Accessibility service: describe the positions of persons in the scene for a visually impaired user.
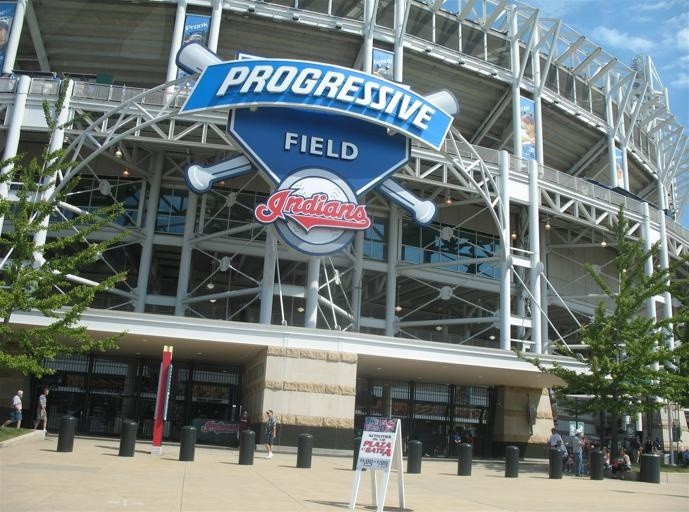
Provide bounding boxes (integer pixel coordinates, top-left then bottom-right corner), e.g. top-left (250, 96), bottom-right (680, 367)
top-left (683, 448), bottom-right (689, 463)
top-left (545, 428), bottom-right (563, 450)
top-left (581, 435), bottom-right (590, 458)
top-left (264, 409), bottom-right (276, 457)
top-left (1, 389), bottom-right (23, 430)
top-left (466, 433), bottom-right (474, 444)
top-left (453, 431), bottom-right (461, 458)
top-left (564, 448), bottom-right (574, 472)
top-left (561, 435), bottom-right (569, 469)
top-left (34, 388), bottom-right (51, 433)
top-left (592, 434), bottom-right (662, 472)
top-left (573, 430), bottom-right (585, 476)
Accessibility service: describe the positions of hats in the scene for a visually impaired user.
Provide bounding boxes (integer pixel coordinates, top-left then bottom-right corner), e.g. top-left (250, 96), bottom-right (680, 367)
top-left (574, 430), bottom-right (582, 436)
